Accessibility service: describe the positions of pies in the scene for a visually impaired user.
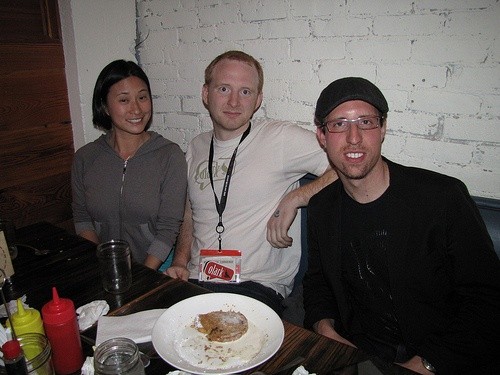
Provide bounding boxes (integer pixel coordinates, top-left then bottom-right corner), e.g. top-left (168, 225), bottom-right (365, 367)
top-left (198, 310), bottom-right (248, 343)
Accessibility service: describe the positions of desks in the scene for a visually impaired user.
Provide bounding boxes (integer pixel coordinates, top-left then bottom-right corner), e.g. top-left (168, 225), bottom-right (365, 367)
top-left (0, 220), bottom-right (428, 375)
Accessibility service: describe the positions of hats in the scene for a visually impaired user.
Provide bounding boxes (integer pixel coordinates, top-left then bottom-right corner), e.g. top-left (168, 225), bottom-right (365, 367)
top-left (314, 76), bottom-right (388, 125)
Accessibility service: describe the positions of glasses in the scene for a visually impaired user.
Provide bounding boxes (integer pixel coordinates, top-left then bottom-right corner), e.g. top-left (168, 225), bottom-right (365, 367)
top-left (319, 116), bottom-right (380, 133)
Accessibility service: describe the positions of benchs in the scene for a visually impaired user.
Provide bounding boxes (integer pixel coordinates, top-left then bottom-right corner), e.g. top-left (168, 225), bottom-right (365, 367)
top-left (291, 173), bottom-right (500, 328)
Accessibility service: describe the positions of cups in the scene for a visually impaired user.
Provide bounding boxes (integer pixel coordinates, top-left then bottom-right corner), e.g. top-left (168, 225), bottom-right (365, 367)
top-left (0, 223), bottom-right (17, 259)
top-left (96, 239), bottom-right (132, 294)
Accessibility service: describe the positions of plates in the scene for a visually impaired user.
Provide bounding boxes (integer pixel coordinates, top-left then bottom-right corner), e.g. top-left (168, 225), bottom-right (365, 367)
top-left (151, 293), bottom-right (284, 375)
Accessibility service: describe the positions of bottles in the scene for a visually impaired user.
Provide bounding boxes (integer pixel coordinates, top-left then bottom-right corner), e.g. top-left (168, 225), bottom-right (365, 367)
top-left (6, 299), bottom-right (55, 375)
top-left (41, 287), bottom-right (83, 375)
top-left (14, 333), bottom-right (55, 375)
top-left (94, 337), bottom-right (144, 375)
top-left (2, 340), bottom-right (28, 375)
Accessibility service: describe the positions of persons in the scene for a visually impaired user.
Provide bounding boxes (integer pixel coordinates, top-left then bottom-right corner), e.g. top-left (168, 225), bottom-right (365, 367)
top-left (163, 50), bottom-right (339, 308)
top-left (70, 59), bottom-right (187, 272)
top-left (302, 76), bottom-right (500, 375)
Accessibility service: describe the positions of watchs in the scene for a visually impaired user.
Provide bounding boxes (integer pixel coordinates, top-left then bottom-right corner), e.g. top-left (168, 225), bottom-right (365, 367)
top-left (422, 358), bottom-right (437, 373)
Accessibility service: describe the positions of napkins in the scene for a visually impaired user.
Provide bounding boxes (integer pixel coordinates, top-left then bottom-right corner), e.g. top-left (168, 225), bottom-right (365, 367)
top-left (93, 306), bottom-right (167, 349)
